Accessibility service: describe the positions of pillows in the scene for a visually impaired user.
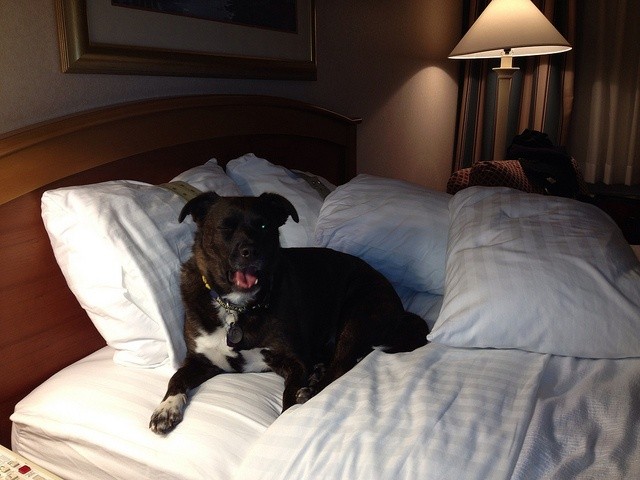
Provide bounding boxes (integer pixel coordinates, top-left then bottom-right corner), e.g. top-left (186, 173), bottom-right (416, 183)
top-left (226, 152), bottom-right (337, 250)
top-left (425, 183), bottom-right (640, 360)
top-left (317, 171), bottom-right (456, 297)
top-left (37, 155), bottom-right (245, 371)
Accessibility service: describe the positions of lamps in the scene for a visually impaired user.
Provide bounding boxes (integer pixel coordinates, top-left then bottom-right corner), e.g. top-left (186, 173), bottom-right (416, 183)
top-left (446, 0), bottom-right (574, 163)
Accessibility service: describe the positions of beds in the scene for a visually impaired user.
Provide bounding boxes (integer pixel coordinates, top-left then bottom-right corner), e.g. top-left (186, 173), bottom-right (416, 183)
top-left (2, 96), bottom-right (640, 480)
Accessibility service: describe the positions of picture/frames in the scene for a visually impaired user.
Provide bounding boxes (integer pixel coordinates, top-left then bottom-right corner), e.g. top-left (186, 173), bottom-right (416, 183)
top-left (53, 1), bottom-right (319, 84)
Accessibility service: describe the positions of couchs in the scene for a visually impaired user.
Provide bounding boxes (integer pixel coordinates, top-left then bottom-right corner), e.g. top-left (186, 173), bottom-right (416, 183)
top-left (448, 152), bottom-right (583, 197)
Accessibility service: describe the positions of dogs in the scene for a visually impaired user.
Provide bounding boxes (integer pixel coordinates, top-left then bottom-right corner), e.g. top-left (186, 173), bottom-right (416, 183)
top-left (150, 190), bottom-right (429, 436)
